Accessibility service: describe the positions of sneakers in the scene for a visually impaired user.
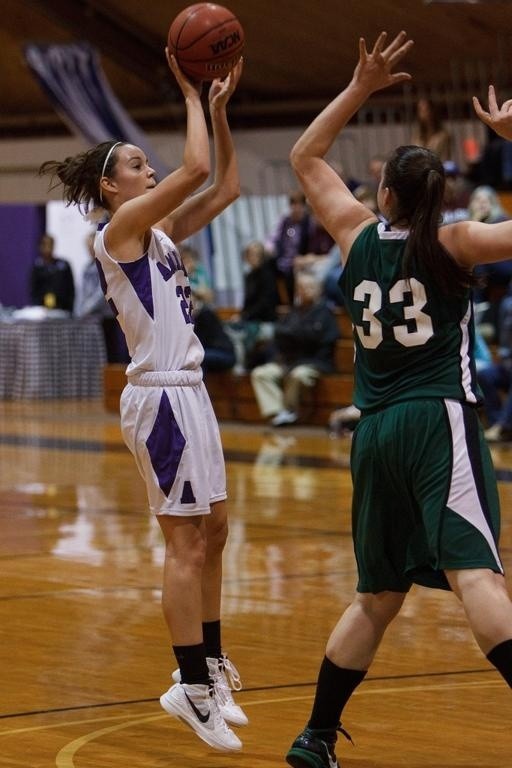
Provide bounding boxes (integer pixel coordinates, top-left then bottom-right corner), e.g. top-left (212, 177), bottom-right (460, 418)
top-left (270, 409), bottom-right (297, 426)
top-left (161, 682), bottom-right (243, 755)
top-left (173, 654), bottom-right (247, 727)
top-left (285, 727), bottom-right (337, 767)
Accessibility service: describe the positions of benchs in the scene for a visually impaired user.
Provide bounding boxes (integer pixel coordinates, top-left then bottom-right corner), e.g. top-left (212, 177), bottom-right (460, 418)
top-left (103, 309), bottom-right (510, 424)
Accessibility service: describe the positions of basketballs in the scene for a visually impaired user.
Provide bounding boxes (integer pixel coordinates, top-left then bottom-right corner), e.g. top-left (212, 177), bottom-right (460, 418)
top-left (169, 2), bottom-right (244, 83)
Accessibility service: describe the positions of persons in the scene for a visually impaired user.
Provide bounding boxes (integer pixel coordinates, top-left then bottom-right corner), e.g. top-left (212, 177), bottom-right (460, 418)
top-left (281, 23), bottom-right (512, 767)
top-left (30, 234), bottom-right (74, 314)
top-left (64, 43), bottom-right (252, 756)
top-left (177, 98), bottom-right (511, 445)
